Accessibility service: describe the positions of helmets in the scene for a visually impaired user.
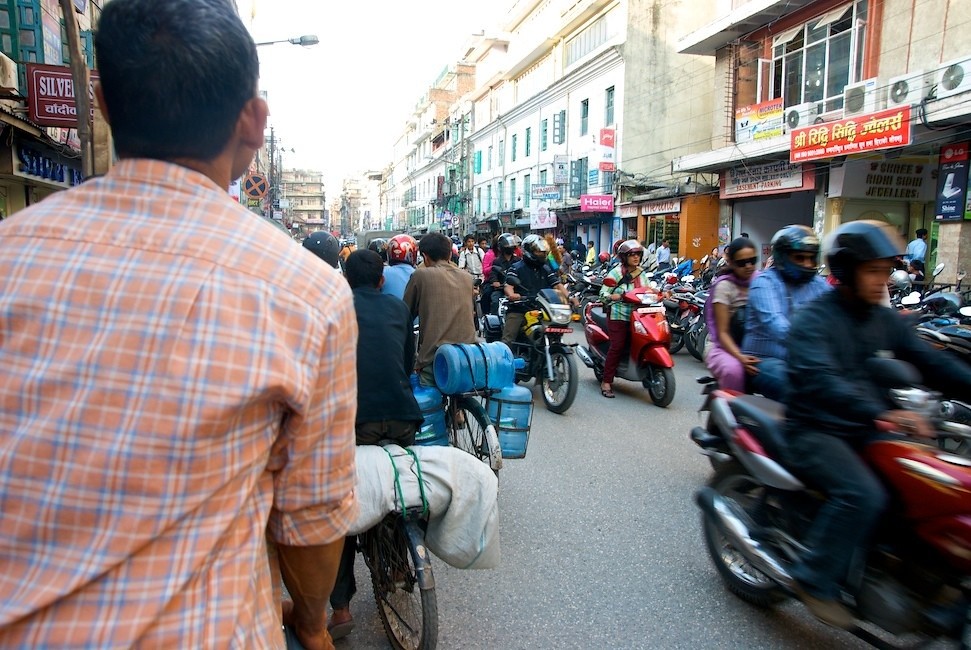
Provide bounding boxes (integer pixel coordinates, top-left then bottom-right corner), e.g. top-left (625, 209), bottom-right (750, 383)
top-left (369, 238), bottom-right (388, 262)
top-left (451, 235), bottom-right (459, 242)
top-left (570, 251), bottom-right (581, 259)
top-left (347, 238), bottom-right (355, 245)
top-left (618, 241), bottom-right (644, 263)
top-left (497, 233), bottom-right (516, 255)
top-left (824, 219), bottom-right (910, 287)
top-left (302, 230), bottom-right (339, 269)
top-left (521, 234), bottom-right (546, 265)
top-left (770, 224), bottom-right (821, 286)
top-left (387, 234), bottom-right (417, 267)
top-left (514, 235), bottom-right (523, 246)
top-left (598, 252), bottom-right (611, 262)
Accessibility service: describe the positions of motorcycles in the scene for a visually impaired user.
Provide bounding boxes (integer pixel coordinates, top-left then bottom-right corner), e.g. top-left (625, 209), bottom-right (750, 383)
top-left (635, 254), bottom-right (720, 360)
top-left (562, 251), bottom-right (621, 313)
top-left (890, 263), bottom-right (971, 354)
top-left (690, 353), bottom-right (971, 650)
top-left (484, 274), bottom-right (582, 416)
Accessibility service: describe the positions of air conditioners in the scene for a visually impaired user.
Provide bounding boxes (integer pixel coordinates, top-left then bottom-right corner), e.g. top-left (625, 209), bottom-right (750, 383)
top-left (843, 77), bottom-right (882, 118)
top-left (0, 52), bottom-right (20, 95)
top-left (785, 102), bottom-right (818, 133)
top-left (936, 56), bottom-right (971, 99)
top-left (888, 70), bottom-right (934, 108)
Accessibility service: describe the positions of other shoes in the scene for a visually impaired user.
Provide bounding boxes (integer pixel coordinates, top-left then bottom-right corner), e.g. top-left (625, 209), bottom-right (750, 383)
top-left (793, 582), bottom-right (855, 632)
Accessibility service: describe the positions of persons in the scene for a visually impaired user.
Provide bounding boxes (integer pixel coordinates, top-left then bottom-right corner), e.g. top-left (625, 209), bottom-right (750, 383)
top-left (302, 229), bottom-right (930, 549)
top-left (328, 249), bottom-right (425, 634)
top-left (0, 0), bottom-right (357, 650)
top-left (786, 219), bottom-right (971, 630)
top-left (741, 224), bottom-right (835, 403)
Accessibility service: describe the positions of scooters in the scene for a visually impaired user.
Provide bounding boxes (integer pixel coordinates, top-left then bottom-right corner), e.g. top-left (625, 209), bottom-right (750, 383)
top-left (576, 273), bottom-right (679, 408)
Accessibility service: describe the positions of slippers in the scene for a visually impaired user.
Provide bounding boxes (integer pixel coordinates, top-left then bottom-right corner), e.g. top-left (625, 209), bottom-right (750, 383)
top-left (327, 620), bottom-right (356, 641)
top-left (601, 387), bottom-right (615, 398)
top-left (445, 409), bottom-right (465, 431)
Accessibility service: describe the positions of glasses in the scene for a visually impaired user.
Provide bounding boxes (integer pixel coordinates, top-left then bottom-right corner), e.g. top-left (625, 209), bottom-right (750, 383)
top-left (795, 254), bottom-right (816, 263)
top-left (627, 253), bottom-right (642, 257)
top-left (731, 256), bottom-right (757, 268)
top-left (860, 265), bottom-right (893, 277)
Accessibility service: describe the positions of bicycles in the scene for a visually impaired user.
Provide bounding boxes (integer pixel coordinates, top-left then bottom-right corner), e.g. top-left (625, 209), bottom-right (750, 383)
top-left (404, 324), bottom-right (534, 491)
top-left (339, 439), bottom-right (438, 650)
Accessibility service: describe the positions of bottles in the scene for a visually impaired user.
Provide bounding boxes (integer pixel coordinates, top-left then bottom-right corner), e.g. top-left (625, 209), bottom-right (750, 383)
top-left (410, 374), bottom-right (449, 446)
top-left (483, 383), bottom-right (532, 456)
top-left (433, 341), bottom-right (525, 395)
